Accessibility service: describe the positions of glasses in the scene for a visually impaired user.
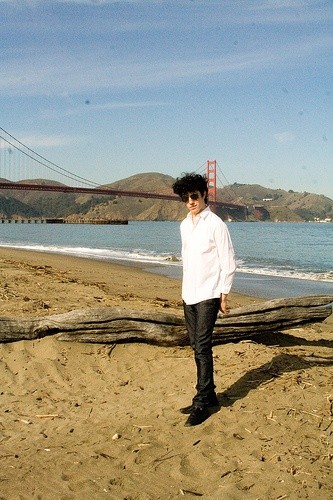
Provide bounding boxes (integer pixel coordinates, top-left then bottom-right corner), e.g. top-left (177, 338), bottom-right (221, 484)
top-left (182, 193), bottom-right (200, 202)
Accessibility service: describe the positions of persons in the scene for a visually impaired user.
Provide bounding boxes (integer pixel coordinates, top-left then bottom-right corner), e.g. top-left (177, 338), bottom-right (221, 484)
top-left (170, 173), bottom-right (237, 426)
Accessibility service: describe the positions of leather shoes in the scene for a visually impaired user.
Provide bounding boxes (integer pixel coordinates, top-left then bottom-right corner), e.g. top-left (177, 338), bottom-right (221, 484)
top-left (184, 408), bottom-right (210, 427)
top-left (180, 400), bottom-right (219, 414)
